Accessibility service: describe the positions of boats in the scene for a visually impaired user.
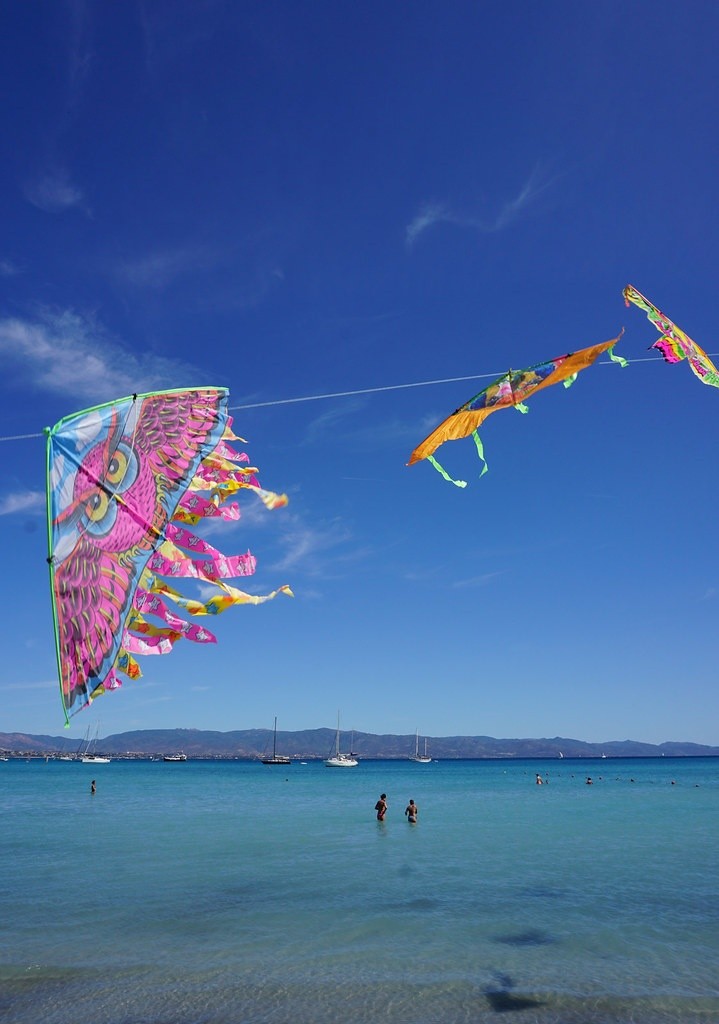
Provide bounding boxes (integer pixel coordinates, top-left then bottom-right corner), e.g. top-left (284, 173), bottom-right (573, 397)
top-left (163, 753), bottom-right (188, 761)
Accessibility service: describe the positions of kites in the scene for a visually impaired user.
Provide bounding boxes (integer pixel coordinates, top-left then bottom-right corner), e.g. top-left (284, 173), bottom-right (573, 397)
top-left (406, 326), bottom-right (632, 488)
top-left (619, 282), bottom-right (718, 392)
top-left (47, 386), bottom-right (293, 729)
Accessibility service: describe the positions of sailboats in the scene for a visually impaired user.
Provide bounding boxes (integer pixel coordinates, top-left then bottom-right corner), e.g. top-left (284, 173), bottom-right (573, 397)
top-left (407, 727), bottom-right (432, 764)
top-left (260, 716), bottom-right (291, 765)
top-left (325, 709), bottom-right (358, 767)
top-left (71, 721), bottom-right (111, 763)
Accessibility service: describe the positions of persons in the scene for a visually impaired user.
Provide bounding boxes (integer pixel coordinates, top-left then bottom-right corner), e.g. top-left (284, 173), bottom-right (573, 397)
top-left (374, 793), bottom-right (388, 821)
top-left (535, 774), bottom-right (550, 785)
top-left (586, 776), bottom-right (594, 785)
top-left (405, 799), bottom-right (419, 823)
top-left (90, 779), bottom-right (98, 796)
